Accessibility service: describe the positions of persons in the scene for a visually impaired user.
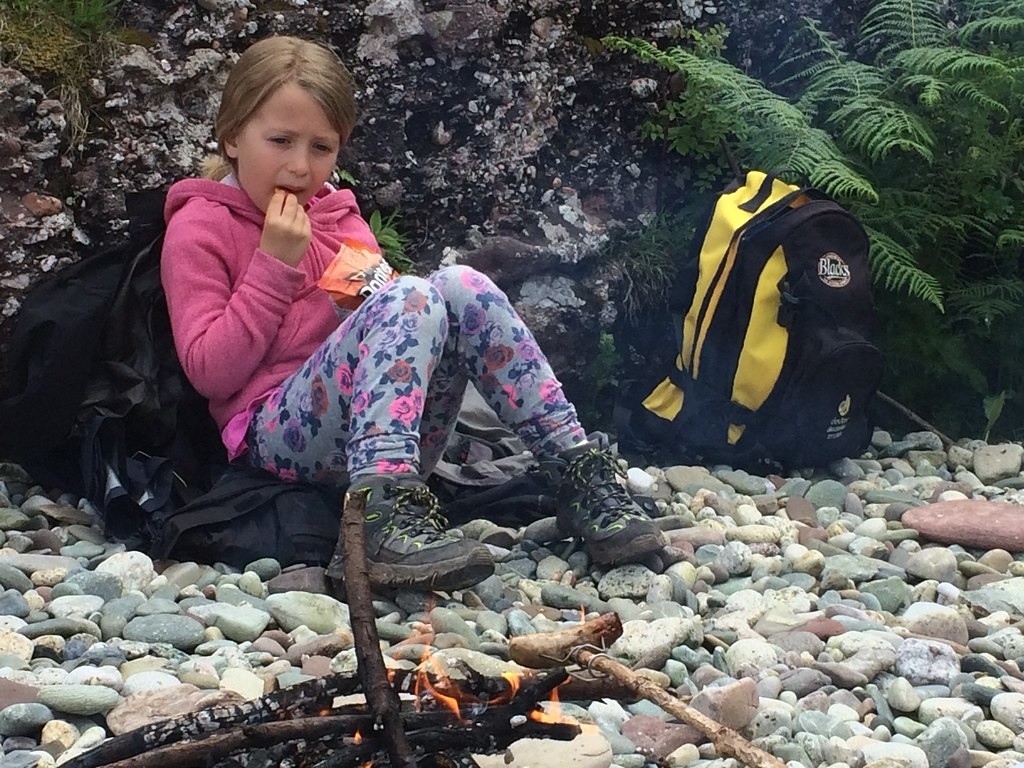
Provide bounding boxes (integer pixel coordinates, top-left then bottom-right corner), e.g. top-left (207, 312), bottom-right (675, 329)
top-left (159, 35), bottom-right (666, 598)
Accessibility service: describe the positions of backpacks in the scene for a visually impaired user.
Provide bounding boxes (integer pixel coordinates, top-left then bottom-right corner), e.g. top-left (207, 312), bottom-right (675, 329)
top-left (628, 160), bottom-right (883, 477)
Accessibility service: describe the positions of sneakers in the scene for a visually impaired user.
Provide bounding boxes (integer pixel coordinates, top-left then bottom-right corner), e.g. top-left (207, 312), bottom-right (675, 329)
top-left (324, 473), bottom-right (495, 591)
top-left (537, 431), bottom-right (667, 567)
